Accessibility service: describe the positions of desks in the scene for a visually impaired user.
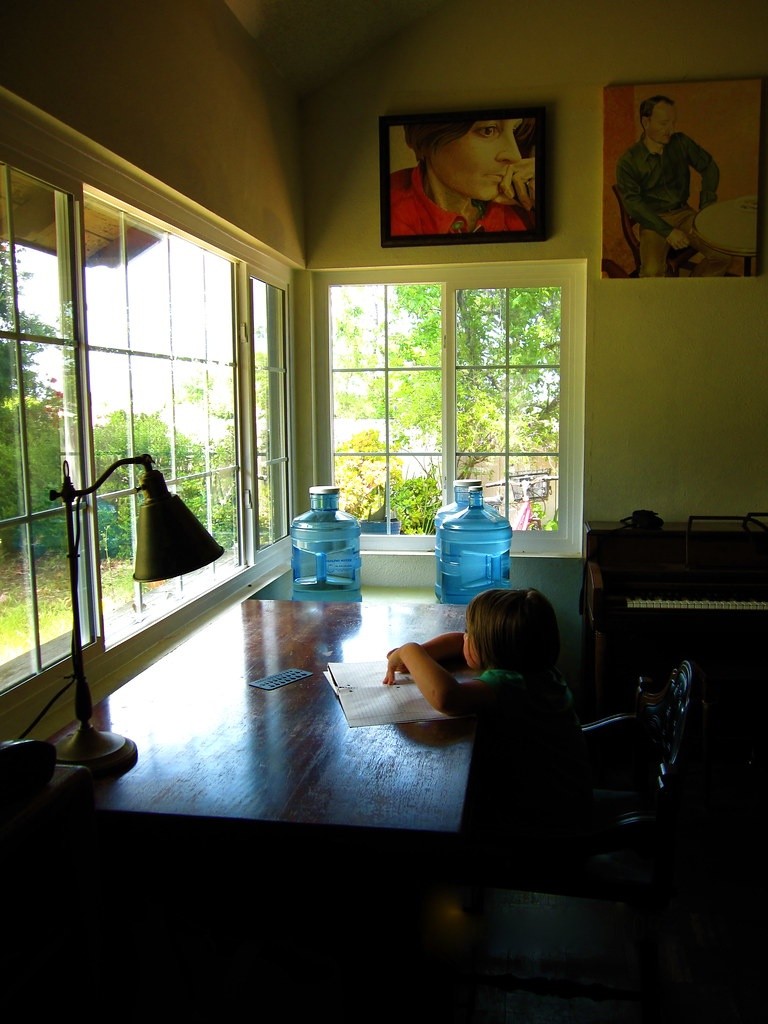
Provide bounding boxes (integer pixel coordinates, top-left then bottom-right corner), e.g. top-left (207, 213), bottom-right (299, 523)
top-left (44, 599), bottom-right (475, 835)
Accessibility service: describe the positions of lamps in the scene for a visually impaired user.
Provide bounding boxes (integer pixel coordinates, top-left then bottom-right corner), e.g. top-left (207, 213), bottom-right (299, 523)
top-left (50, 454), bottom-right (224, 777)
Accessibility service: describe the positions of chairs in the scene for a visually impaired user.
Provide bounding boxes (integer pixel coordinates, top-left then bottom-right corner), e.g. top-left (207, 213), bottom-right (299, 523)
top-left (468, 659), bottom-right (712, 1024)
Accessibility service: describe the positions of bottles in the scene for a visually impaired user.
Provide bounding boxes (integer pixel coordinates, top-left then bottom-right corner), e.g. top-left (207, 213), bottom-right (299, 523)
top-left (435, 480), bottom-right (512, 605)
top-left (291, 485), bottom-right (362, 602)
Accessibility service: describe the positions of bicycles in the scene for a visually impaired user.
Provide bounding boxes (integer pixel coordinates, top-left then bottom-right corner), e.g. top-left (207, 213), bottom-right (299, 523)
top-left (479, 466), bottom-right (559, 531)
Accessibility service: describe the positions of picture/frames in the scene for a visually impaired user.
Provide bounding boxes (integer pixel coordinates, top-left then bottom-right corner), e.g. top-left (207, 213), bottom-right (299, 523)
top-left (379, 107), bottom-right (547, 249)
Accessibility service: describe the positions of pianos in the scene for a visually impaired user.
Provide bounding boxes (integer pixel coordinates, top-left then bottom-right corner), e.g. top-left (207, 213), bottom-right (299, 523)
top-left (583, 512), bottom-right (767, 695)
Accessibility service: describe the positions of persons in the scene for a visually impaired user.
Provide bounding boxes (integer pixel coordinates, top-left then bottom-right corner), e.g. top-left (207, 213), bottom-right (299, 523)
top-left (407, 586), bottom-right (593, 826)
